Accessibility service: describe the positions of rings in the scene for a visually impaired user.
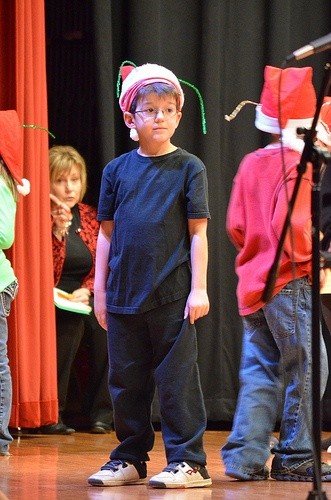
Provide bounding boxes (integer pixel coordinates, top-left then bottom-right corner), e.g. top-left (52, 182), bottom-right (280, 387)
top-left (57, 209), bottom-right (60, 215)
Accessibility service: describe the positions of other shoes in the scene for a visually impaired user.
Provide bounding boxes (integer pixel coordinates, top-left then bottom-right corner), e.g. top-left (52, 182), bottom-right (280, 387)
top-left (40, 421), bottom-right (71, 434)
top-left (85, 426), bottom-right (108, 435)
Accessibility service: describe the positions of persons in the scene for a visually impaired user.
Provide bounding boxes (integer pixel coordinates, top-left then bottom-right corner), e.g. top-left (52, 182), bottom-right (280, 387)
top-left (223, 64), bottom-right (331, 482)
top-left (30, 144), bottom-right (112, 434)
top-left (0, 110), bottom-right (30, 456)
top-left (86, 60), bottom-right (212, 489)
top-left (308, 95), bottom-right (331, 455)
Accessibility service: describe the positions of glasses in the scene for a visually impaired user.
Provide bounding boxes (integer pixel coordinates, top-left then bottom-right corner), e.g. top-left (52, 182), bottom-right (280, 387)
top-left (133, 106), bottom-right (176, 119)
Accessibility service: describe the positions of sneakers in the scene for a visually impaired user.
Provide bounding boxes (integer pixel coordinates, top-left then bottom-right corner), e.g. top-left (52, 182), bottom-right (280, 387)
top-left (89, 459), bottom-right (148, 487)
top-left (151, 456), bottom-right (212, 488)
top-left (225, 466), bottom-right (268, 480)
top-left (272, 458), bottom-right (331, 484)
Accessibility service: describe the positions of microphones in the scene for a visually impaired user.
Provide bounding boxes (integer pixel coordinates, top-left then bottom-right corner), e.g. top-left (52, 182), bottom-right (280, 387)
top-left (285, 33), bottom-right (331, 63)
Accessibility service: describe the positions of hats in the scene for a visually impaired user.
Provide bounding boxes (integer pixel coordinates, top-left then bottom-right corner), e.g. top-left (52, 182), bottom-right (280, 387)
top-left (254, 66), bottom-right (316, 146)
top-left (0, 110), bottom-right (30, 195)
top-left (314, 98), bottom-right (331, 145)
top-left (119, 62), bottom-right (185, 114)
